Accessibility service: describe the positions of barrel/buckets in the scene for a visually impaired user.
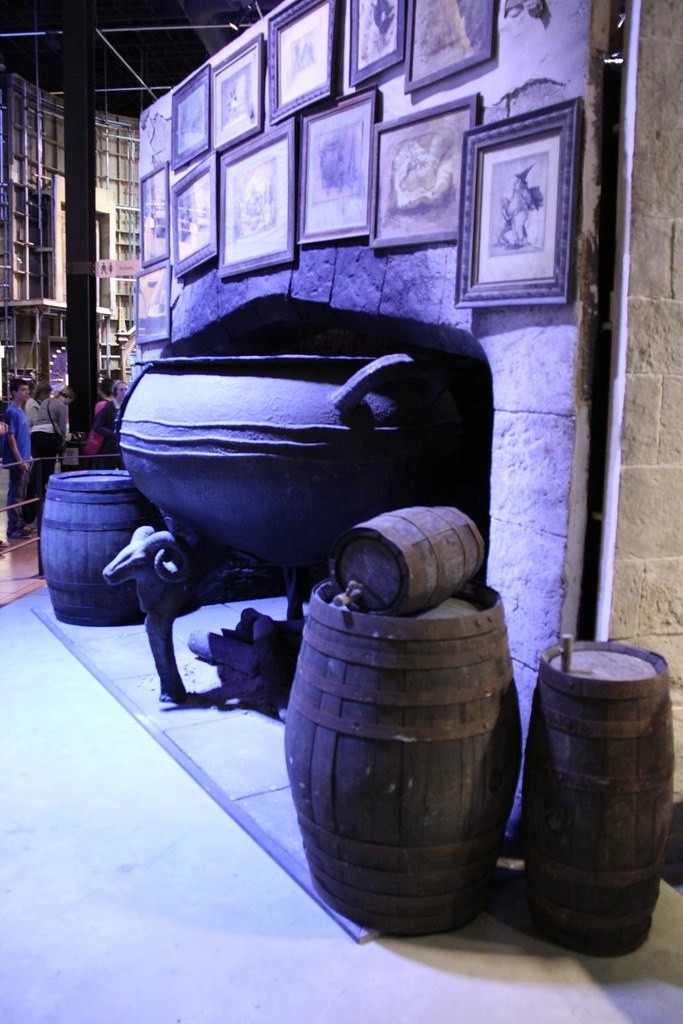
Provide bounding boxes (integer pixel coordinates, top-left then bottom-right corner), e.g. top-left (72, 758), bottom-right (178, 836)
top-left (41, 470), bottom-right (148, 627)
top-left (285, 578), bottom-right (522, 937)
top-left (521, 633), bottom-right (675, 958)
top-left (330, 507), bottom-right (485, 616)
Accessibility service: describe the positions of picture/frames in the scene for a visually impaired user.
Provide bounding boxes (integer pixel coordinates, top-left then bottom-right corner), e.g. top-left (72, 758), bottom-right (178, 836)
top-left (349, 0), bottom-right (407, 89)
top-left (210, 33), bottom-right (265, 153)
top-left (294, 82), bottom-right (378, 246)
top-left (139, 160), bottom-right (171, 270)
top-left (267, 0), bottom-right (341, 125)
top-left (170, 63), bottom-right (211, 171)
top-left (454, 96), bottom-right (585, 308)
top-left (136, 259), bottom-right (171, 345)
top-left (404, 0), bottom-right (496, 95)
top-left (368, 93), bottom-right (477, 250)
top-left (172, 150), bottom-right (219, 280)
top-left (218, 116), bottom-right (295, 277)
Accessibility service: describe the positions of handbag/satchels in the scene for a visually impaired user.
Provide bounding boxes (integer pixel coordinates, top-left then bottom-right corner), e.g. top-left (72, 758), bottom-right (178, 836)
top-left (56, 432), bottom-right (64, 459)
top-left (85, 430), bottom-right (104, 453)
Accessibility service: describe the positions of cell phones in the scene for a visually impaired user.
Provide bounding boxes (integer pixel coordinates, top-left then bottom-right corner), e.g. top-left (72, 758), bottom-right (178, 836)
top-left (26, 467), bottom-right (31, 474)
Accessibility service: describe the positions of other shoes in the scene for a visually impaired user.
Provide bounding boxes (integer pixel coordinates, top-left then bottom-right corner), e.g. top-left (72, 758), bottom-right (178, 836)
top-left (6, 526), bottom-right (38, 540)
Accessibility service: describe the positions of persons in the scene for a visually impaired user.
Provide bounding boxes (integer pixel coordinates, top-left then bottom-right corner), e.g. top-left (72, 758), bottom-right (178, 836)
top-left (0, 376), bottom-right (129, 556)
